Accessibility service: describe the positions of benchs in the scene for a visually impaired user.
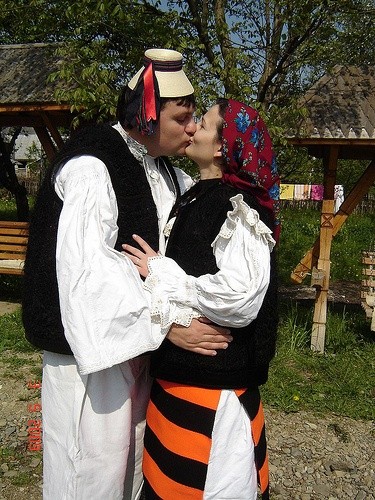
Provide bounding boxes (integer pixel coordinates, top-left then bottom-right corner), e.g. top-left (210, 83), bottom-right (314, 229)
top-left (0, 221), bottom-right (29, 275)
top-left (361, 251), bottom-right (375, 331)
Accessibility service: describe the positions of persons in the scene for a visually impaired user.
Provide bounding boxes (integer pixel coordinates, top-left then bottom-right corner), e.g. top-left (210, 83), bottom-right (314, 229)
top-left (19, 49), bottom-right (234, 500)
top-left (119, 97), bottom-right (284, 499)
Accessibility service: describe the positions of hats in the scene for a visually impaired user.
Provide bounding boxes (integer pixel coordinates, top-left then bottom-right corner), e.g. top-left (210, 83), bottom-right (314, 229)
top-left (128, 47), bottom-right (195, 136)
